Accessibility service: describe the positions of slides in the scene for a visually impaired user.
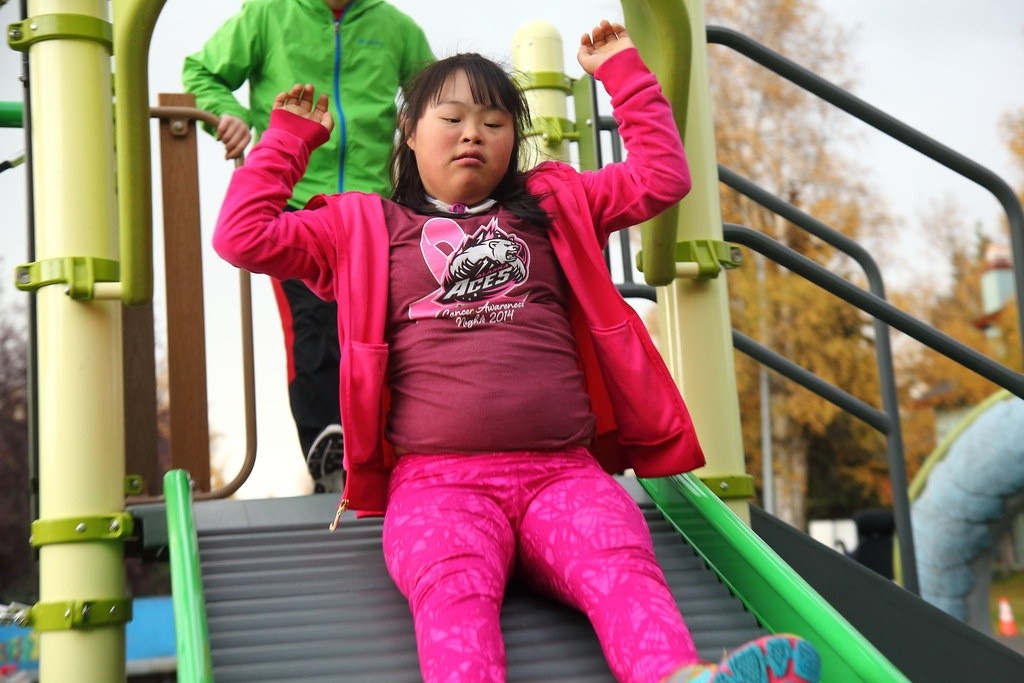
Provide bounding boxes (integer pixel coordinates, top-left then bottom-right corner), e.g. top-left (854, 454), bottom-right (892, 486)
top-left (163, 467), bottom-right (912, 683)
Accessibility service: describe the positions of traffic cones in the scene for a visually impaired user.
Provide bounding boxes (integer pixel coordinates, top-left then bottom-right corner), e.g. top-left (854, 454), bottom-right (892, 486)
top-left (997, 597), bottom-right (1018, 637)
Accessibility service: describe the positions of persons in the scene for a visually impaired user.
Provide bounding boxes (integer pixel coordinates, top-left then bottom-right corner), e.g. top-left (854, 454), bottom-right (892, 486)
top-left (212, 20), bottom-right (822, 683)
top-left (183, 0), bottom-right (437, 494)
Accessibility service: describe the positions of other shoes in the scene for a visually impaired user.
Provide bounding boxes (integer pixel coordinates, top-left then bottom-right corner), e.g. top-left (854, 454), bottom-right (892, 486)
top-left (308, 424), bottom-right (347, 493)
top-left (688, 633), bottom-right (820, 683)
